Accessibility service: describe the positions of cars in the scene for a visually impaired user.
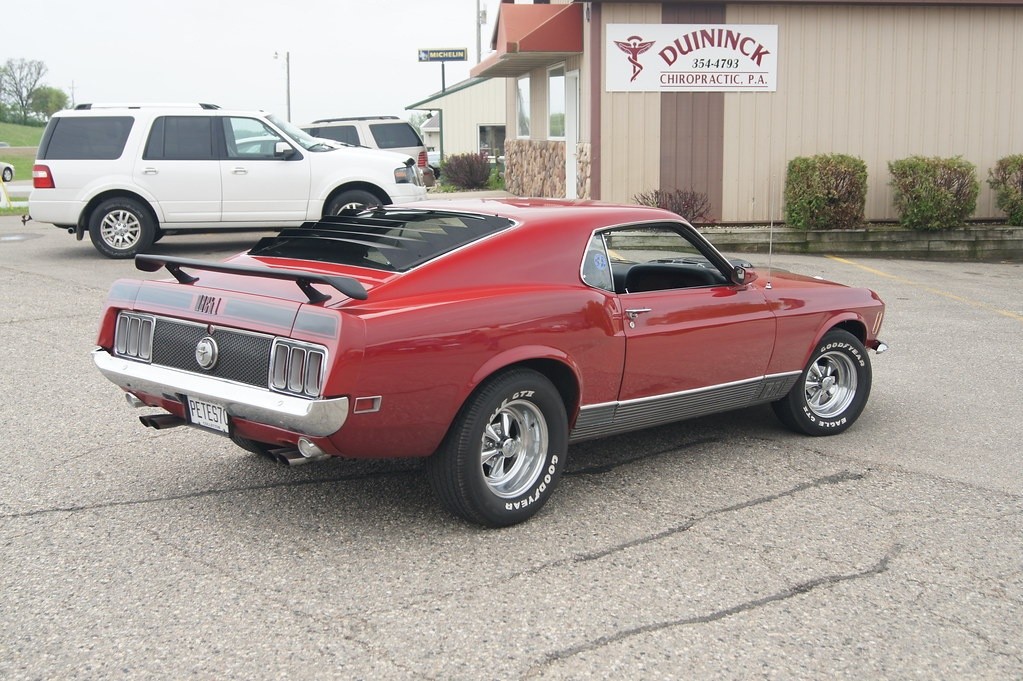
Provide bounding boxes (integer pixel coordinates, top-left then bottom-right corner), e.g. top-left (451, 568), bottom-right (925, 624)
top-left (229, 132), bottom-right (294, 153)
top-left (479, 147), bottom-right (505, 164)
top-left (0, 161), bottom-right (15, 182)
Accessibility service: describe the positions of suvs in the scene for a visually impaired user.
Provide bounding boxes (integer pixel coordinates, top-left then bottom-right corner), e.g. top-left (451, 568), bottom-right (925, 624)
top-left (295, 114), bottom-right (437, 194)
top-left (21, 101), bottom-right (430, 260)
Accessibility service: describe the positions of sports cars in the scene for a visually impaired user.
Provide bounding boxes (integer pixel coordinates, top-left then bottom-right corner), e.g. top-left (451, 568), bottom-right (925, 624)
top-left (98, 197), bottom-right (888, 530)
